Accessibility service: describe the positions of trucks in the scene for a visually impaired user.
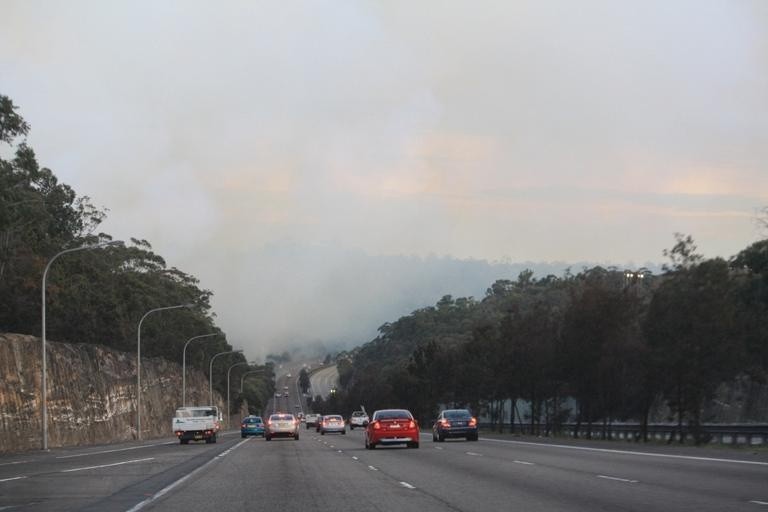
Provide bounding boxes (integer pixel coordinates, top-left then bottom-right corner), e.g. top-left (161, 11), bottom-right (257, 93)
top-left (170, 405), bottom-right (224, 445)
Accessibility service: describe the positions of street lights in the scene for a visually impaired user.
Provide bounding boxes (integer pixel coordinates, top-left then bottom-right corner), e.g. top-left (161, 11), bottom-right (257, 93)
top-left (180, 326), bottom-right (266, 432)
top-left (136, 301), bottom-right (197, 445)
top-left (43, 236), bottom-right (124, 454)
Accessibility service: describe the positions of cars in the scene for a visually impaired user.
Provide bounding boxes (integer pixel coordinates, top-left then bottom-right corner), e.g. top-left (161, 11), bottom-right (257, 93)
top-left (432, 409), bottom-right (480, 443)
top-left (240, 359), bottom-right (346, 442)
top-left (364, 408), bottom-right (420, 449)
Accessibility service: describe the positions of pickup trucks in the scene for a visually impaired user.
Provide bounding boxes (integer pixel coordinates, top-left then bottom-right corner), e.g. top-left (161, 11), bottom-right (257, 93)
top-left (349, 410), bottom-right (370, 430)
